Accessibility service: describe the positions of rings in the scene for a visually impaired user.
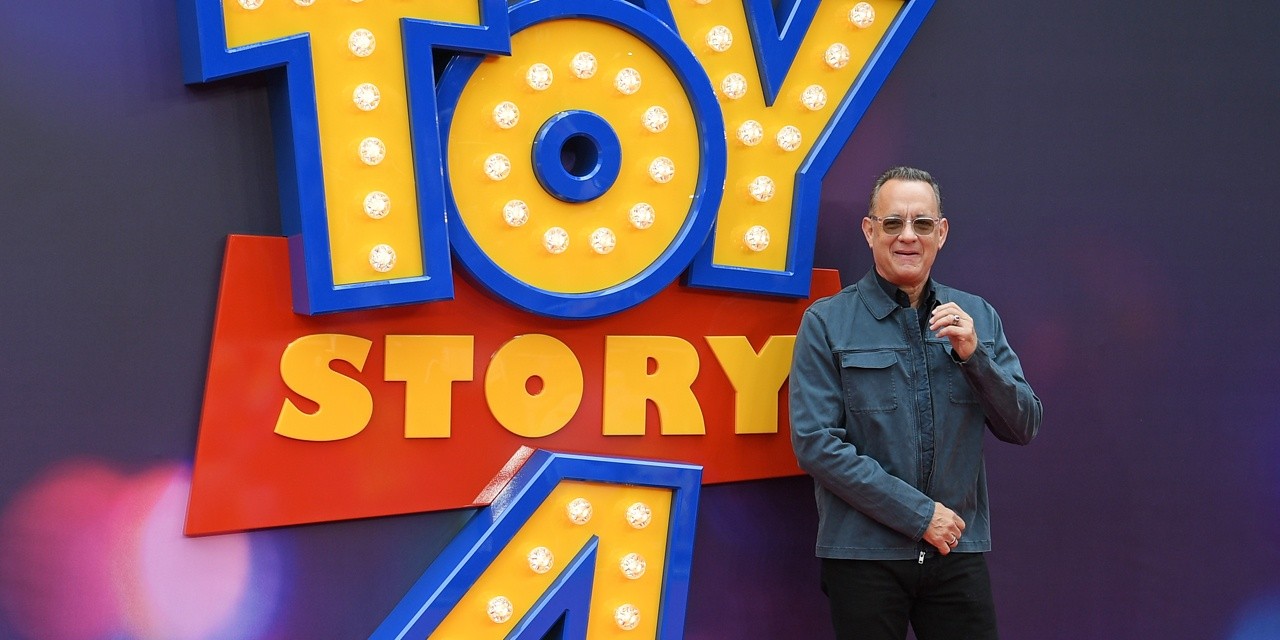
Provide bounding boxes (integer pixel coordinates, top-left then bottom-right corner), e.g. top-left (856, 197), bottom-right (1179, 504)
top-left (949, 537), bottom-right (956, 545)
top-left (952, 314), bottom-right (960, 326)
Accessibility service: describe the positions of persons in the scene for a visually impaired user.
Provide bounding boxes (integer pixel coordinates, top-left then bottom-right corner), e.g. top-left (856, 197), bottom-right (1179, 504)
top-left (789, 165), bottom-right (1043, 640)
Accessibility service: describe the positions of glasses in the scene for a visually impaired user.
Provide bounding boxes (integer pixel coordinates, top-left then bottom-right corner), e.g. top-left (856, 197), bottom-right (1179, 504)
top-left (869, 214), bottom-right (942, 234)
